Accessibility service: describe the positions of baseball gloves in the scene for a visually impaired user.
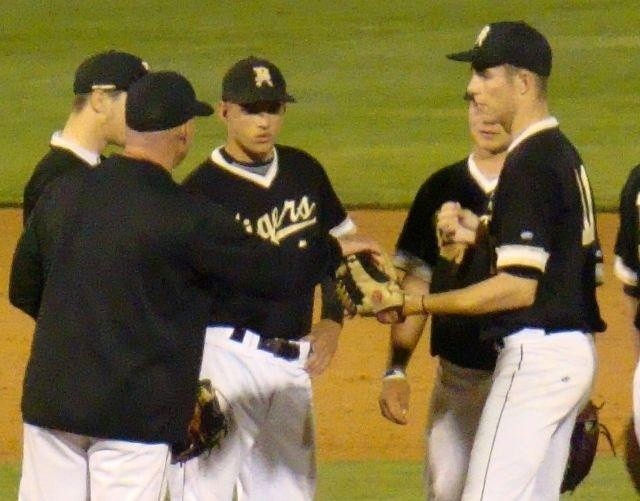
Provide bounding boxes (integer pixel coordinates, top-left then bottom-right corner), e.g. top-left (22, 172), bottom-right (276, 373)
top-left (331, 255), bottom-right (407, 325)
top-left (170, 379), bottom-right (228, 466)
top-left (562, 400), bottom-right (598, 496)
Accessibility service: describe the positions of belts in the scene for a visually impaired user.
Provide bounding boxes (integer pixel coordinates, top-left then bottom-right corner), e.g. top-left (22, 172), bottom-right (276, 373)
top-left (445, 356), bottom-right (496, 373)
top-left (231, 327), bottom-right (299, 362)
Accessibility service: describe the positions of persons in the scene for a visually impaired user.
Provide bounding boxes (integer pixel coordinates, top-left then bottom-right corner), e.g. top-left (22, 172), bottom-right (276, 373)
top-left (609, 164), bottom-right (640, 457)
top-left (398, 19), bottom-right (607, 501)
top-left (7, 71), bottom-right (382, 501)
top-left (17, 49), bottom-right (145, 231)
top-left (167, 56), bottom-right (357, 501)
top-left (378, 91), bottom-right (504, 501)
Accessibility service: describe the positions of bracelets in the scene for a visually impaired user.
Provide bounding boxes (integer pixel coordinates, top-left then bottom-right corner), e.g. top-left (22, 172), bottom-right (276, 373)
top-left (381, 370), bottom-right (407, 380)
top-left (389, 345), bottom-right (411, 369)
top-left (422, 294), bottom-right (429, 315)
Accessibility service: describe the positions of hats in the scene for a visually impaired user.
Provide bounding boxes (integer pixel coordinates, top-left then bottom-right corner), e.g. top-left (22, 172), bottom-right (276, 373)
top-left (222, 55), bottom-right (296, 107)
top-left (125, 70), bottom-right (216, 133)
top-left (73, 50), bottom-right (152, 93)
top-left (445, 21), bottom-right (552, 77)
